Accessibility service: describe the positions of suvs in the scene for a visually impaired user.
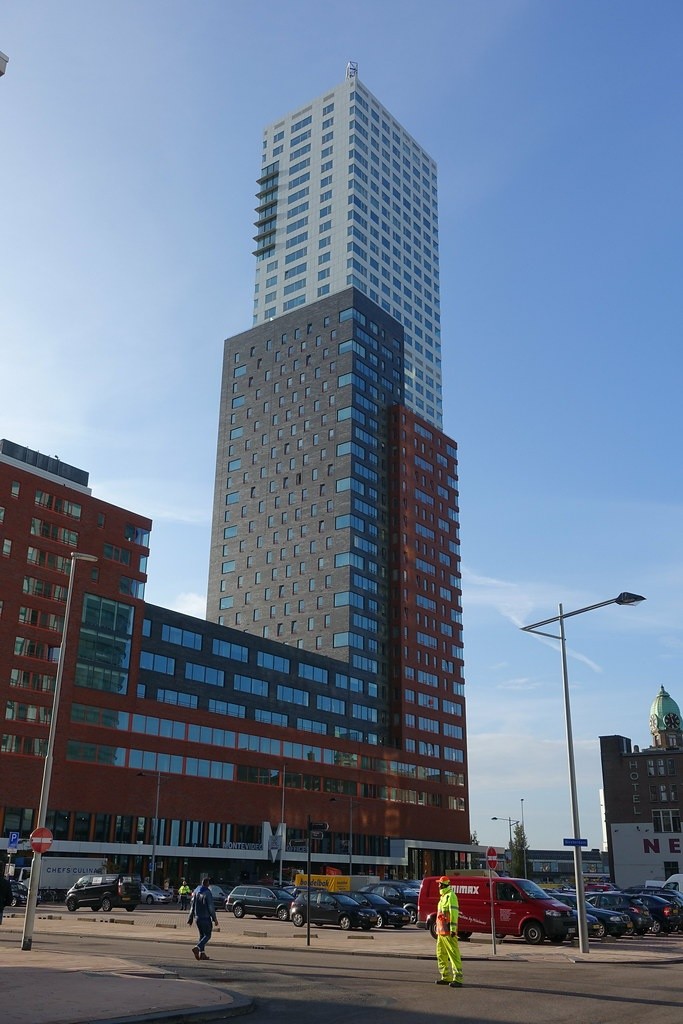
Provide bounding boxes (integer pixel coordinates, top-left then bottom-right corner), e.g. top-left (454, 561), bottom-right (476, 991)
top-left (64, 873), bottom-right (142, 912)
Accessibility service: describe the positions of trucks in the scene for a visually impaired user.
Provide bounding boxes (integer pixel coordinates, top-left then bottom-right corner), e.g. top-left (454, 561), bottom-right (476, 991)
top-left (19, 855), bottom-right (110, 902)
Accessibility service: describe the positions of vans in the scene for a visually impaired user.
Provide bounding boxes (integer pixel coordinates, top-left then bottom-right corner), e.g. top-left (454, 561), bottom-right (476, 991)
top-left (415, 874), bottom-right (576, 945)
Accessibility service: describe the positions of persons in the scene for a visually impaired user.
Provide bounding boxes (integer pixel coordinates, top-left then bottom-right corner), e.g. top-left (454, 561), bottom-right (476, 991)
top-left (187, 878), bottom-right (217, 961)
top-left (435, 876), bottom-right (463, 987)
top-left (178, 881), bottom-right (190, 911)
top-left (164, 881), bottom-right (169, 891)
top-left (0, 861), bottom-right (14, 924)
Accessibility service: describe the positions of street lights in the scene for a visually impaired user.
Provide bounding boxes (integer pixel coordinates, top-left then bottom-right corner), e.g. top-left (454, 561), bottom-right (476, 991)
top-left (136, 771), bottom-right (170, 884)
top-left (17, 552), bottom-right (99, 952)
top-left (519, 591), bottom-right (647, 955)
top-left (329, 796), bottom-right (362, 877)
top-left (491, 816), bottom-right (521, 877)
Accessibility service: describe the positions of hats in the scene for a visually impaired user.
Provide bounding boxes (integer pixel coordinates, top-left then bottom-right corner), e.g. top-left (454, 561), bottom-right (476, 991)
top-left (436, 876), bottom-right (449, 885)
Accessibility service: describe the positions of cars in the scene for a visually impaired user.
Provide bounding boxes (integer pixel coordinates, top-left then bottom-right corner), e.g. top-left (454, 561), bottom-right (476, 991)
top-left (289, 890), bottom-right (379, 932)
top-left (140, 882), bottom-right (172, 906)
top-left (9, 880), bottom-right (42, 907)
top-left (189, 884), bottom-right (236, 911)
top-left (225, 884), bottom-right (297, 922)
top-left (280, 873), bottom-right (422, 929)
top-left (539, 873), bottom-right (683, 942)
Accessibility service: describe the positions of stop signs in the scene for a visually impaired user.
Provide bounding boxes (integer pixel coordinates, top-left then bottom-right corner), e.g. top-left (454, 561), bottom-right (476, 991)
top-left (29, 827), bottom-right (53, 853)
top-left (486, 846), bottom-right (498, 870)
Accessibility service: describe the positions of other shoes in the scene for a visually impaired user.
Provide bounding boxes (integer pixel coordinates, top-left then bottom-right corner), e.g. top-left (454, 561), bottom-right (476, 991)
top-left (434, 980), bottom-right (449, 985)
top-left (199, 953), bottom-right (209, 960)
top-left (191, 947), bottom-right (198, 960)
top-left (449, 982), bottom-right (462, 987)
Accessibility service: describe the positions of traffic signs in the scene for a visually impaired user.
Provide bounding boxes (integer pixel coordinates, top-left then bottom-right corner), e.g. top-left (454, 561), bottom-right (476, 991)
top-left (311, 822), bottom-right (329, 831)
top-left (289, 838), bottom-right (307, 847)
top-left (311, 831), bottom-right (323, 840)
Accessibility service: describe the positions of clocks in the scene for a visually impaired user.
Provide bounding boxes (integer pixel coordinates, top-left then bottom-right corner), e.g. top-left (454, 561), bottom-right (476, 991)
top-left (649, 714), bottom-right (659, 730)
top-left (663, 712), bottom-right (681, 730)
